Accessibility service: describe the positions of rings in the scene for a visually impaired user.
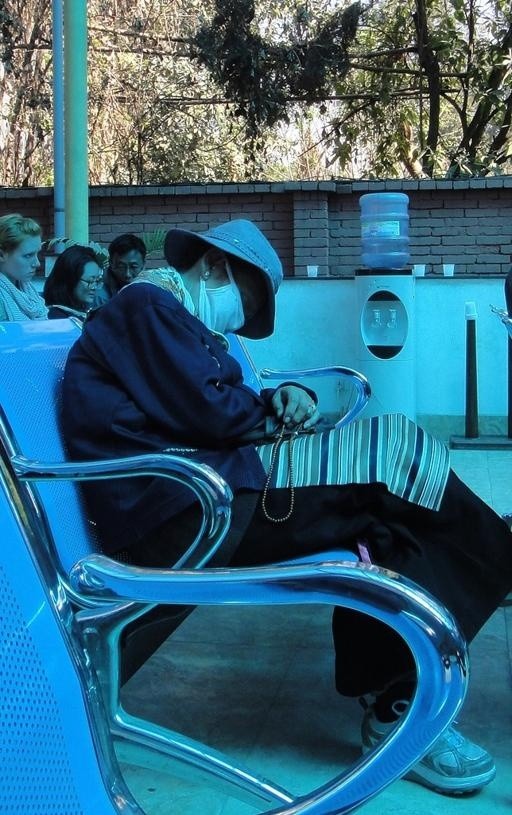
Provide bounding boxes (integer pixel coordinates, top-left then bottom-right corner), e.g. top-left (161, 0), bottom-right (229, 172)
top-left (308, 403), bottom-right (317, 412)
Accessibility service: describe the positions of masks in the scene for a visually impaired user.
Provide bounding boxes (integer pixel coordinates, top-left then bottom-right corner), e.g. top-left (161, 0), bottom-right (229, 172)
top-left (198, 257), bottom-right (245, 335)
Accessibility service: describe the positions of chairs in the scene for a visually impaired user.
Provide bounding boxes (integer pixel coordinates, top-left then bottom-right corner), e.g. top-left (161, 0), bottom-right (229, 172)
top-left (0, 312), bottom-right (472, 813)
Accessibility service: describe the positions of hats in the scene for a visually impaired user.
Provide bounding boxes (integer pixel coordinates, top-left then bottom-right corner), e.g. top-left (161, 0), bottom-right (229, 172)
top-left (164, 219), bottom-right (283, 339)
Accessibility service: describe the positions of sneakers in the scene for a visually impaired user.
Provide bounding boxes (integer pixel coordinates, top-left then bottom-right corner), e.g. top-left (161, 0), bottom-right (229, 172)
top-left (360, 694), bottom-right (496, 796)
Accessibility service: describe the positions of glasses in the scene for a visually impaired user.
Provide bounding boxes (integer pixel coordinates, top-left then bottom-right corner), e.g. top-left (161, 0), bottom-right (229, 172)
top-left (81, 279), bottom-right (103, 290)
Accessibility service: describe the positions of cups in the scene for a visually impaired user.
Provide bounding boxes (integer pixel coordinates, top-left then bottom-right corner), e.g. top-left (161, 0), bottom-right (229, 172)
top-left (414, 264), bottom-right (426, 277)
top-left (464, 301), bottom-right (478, 321)
top-left (443, 264), bottom-right (455, 277)
top-left (307, 266), bottom-right (317, 277)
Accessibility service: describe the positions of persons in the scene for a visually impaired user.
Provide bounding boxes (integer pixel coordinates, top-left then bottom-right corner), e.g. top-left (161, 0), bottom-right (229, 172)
top-left (59, 220), bottom-right (512, 795)
top-left (0, 212), bottom-right (50, 325)
top-left (40, 244), bottom-right (107, 322)
top-left (93, 234), bottom-right (146, 302)
